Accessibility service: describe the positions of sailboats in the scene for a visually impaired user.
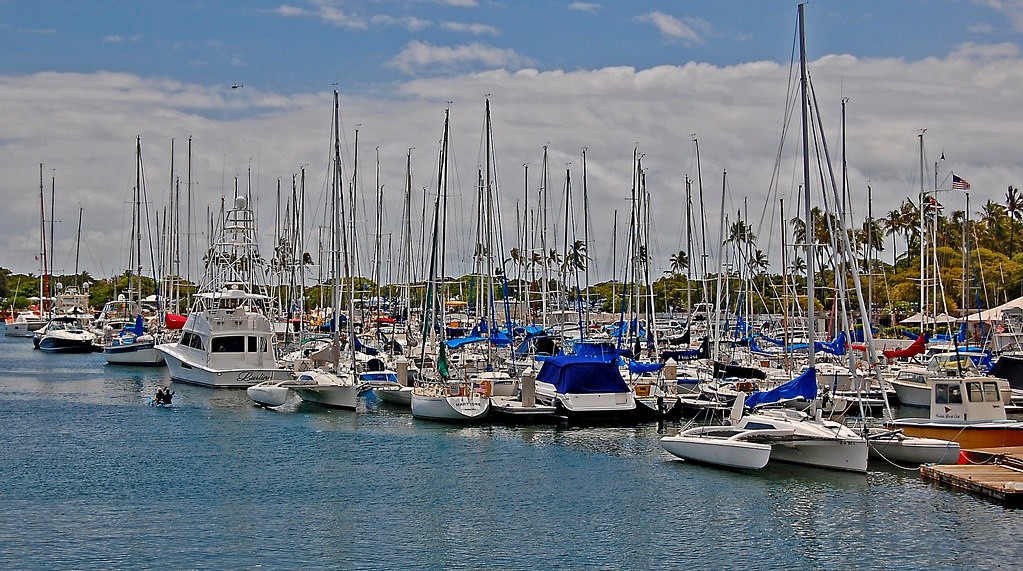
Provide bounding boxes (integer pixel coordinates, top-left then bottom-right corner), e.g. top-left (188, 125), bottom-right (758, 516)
top-left (1, 0), bottom-right (1022, 477)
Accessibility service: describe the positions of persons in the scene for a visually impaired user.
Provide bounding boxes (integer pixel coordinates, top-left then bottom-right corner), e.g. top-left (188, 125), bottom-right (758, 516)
top-left (155, 388), bottom-right (165, 405)
top-left (163, 389), bottom-right (172, 404)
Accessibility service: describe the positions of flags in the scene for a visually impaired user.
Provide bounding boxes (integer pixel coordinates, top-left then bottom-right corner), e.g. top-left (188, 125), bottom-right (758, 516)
top-left (952, 175), bottom-right (970, 190)
top-left (929, 196), bottom-right (943, 210)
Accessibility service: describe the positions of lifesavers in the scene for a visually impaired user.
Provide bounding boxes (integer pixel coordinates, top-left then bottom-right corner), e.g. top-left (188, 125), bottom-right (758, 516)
top-left (479, 381), bottom-right (491, 399)
